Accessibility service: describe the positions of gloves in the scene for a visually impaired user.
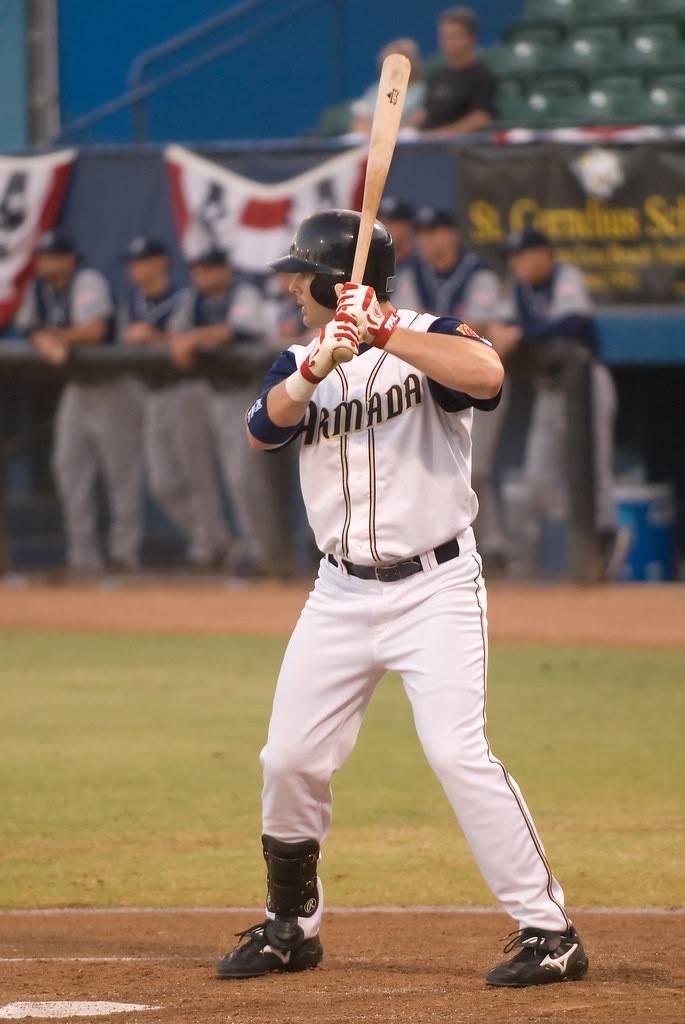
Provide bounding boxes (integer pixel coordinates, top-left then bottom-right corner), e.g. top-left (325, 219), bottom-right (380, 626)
top-left (300, 312), bottom-right (365, 384)
top-left (334, 281), bottom-right (400, 349)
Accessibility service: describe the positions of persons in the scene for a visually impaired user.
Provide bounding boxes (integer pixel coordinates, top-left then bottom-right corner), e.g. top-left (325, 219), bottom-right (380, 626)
top-left (215, 210), bottom-right (590, 987)
top-left (177, 247), bottom-right (291, 575)
top-left (267, 205), bottom-right (631, 579)
top-left (14, 228), bottom-right (147, 582)
top-left (119, 237), bottom-right (233, 573)
top-left (351, 39), bottom-right (427, 133)
top-left (419, 8), bottom-right (494, 139)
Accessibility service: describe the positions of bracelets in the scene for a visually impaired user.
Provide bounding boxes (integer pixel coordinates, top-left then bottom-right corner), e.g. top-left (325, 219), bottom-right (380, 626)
top-left (286, 369), bottom-right (317, 402)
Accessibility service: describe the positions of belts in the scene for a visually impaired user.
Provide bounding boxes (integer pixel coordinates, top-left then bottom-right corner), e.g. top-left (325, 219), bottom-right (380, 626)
top-left (327, 540), bottom-right (459, 582)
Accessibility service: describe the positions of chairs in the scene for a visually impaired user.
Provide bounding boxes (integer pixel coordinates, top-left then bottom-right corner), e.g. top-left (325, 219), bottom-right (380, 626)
top-left (316, 0), bottom-right (685, 136)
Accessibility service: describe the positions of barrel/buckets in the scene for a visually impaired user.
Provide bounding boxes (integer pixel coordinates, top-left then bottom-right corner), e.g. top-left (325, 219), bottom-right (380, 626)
top-left (611, 485), bottom-right (674, 582)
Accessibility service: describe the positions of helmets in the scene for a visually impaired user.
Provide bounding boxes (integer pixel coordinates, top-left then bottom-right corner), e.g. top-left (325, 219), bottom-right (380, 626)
top-left (266, 209), bottom-right (395, 310)
top-left (415, 206), bottom-right (459, 229)
top-left (381, 195), bottom-right (413, 220)
top-left (119, 238), bottom-right (166, 261)
top-left (37, 231), bottom-right (71, 254)
top-left (187, 248), bottom-right (226, 265)
top-left (516, 232), bottom-right (548, 252)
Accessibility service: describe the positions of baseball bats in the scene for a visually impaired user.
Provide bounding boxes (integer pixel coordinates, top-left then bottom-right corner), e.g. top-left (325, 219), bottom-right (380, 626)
top-left (332, 52), bottom-right (412, 363)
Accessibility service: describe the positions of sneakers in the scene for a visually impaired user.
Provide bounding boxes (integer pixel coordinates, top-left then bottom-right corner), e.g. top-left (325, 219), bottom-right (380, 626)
top-left (214, 919), bottom-right (323, 976)
top-left (485, 926), bottom-right (589, 986)
